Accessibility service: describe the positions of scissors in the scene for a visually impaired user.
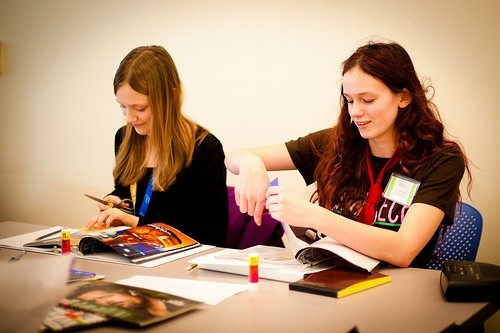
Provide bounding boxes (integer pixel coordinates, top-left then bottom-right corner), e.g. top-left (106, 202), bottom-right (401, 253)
top-left (83, 193), bottom-right (134, 215)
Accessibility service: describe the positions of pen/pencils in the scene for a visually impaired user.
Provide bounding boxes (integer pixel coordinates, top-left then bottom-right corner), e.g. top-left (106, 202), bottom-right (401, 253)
top-left (186, 264), bottom-right (197, 270)
top-left (35, 230), bottom-right (61, 240)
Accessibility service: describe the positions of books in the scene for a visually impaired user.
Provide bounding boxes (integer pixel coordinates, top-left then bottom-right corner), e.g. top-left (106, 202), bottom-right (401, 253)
top-left (229, 236), bottom-right (392, 298)
top-left (0, 223), bottom-right (204, 333)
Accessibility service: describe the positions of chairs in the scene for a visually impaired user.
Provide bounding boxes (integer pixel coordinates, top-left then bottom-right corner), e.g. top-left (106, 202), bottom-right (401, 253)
top-left (226, 186), bottom-right (284, 249)
top-left (425, 201), bottom-right (483, 270)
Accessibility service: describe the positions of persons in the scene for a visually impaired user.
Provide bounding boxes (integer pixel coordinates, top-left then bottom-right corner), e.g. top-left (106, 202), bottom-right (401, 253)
top-left (224, 42), bottom-right (473, 267)
top-left (74, 282), bottom-right (191, 318)
top-left (84, 44), bottom-right (229, 248)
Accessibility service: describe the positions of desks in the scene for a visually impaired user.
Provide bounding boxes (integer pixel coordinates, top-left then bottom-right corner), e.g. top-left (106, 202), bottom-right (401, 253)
top-left (0, 221), bottom-right (500, 333)
